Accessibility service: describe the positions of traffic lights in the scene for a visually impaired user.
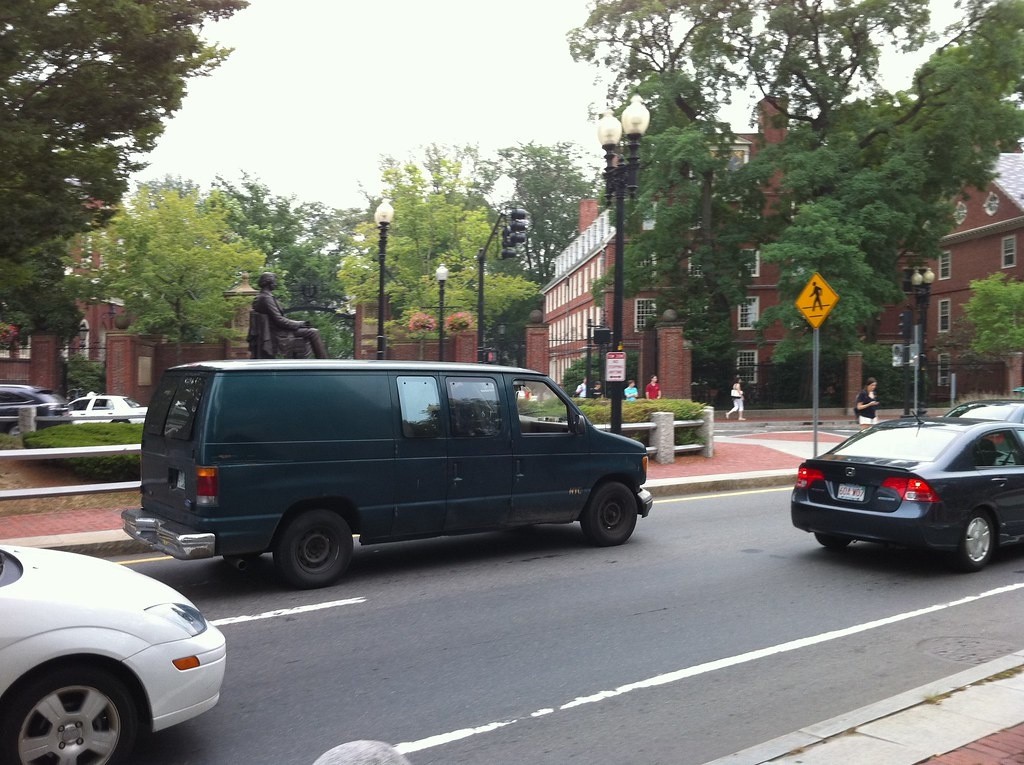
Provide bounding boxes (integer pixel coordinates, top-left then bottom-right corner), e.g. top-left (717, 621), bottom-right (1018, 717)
top-left (501, 227), bottom-right (518, 258)
top-left (899, 309), bottom-right (912, 341)
top-left (511, 207), bottom-right (528, 244)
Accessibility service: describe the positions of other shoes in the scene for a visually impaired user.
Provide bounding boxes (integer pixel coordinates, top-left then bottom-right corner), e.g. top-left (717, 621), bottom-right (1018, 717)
top-left (726, 413), bottom-right (730, 420)
top-left (738, 417), bottom-right (747, 421)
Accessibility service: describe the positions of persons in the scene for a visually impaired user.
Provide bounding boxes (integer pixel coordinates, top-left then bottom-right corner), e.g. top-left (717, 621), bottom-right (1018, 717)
top-left (624, 380), bottom-right (639, 401)
top-left (855, 377), bottom-right (880, 430)
top-left (725, 377), bottom-right (747, 420)
top-left (575, 377), bottom-right (588, 397)
top-left (645, 375), bottom-right (661, 400)
top-left (537, 381), bottom-right (564, 402)
top-left (588, 381), bottom-right (604, 399)
top-left (252, 272), bottom-right (330, 359)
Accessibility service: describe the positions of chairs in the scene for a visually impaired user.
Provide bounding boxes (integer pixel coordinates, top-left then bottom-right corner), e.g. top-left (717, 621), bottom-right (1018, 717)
top-left (973, 441), bottom-right (995, 465)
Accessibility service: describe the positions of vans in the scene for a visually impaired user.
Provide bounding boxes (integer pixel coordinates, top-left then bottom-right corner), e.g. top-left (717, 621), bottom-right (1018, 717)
top-left (120, 359), bottom-right (653, 590)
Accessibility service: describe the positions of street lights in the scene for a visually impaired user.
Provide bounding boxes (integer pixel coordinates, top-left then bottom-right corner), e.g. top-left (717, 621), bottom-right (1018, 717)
top-left (595, 95), bottom-right (651, 433)
top-left (374, 199), bottom-right (394, 359)
top-left (435, 264), bottom-right (448, 362)
top-left (911, 268), bottom-right (935, 414)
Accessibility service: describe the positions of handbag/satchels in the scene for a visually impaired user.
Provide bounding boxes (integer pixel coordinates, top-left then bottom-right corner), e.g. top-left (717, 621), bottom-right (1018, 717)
top-left (730, 389), bottom-right (744, 398)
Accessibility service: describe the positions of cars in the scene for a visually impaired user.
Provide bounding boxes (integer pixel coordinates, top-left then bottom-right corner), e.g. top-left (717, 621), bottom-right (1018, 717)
top-left (944, 399), bottom-right (1024, 451)
top-left (789, 414), bottom-right (1024, 573)
top-left (67, 390), bottom-right (149, 424)
top-left (0, 545), bottom-right (227, 765)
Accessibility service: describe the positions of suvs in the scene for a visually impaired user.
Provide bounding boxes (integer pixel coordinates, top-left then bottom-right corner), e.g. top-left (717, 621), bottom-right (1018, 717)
top-left (0, 382), bottom-right (73, 435)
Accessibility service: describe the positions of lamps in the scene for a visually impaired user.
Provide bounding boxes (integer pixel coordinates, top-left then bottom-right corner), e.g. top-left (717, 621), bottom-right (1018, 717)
top-left (101, 304), bottom-right (117, 319)
